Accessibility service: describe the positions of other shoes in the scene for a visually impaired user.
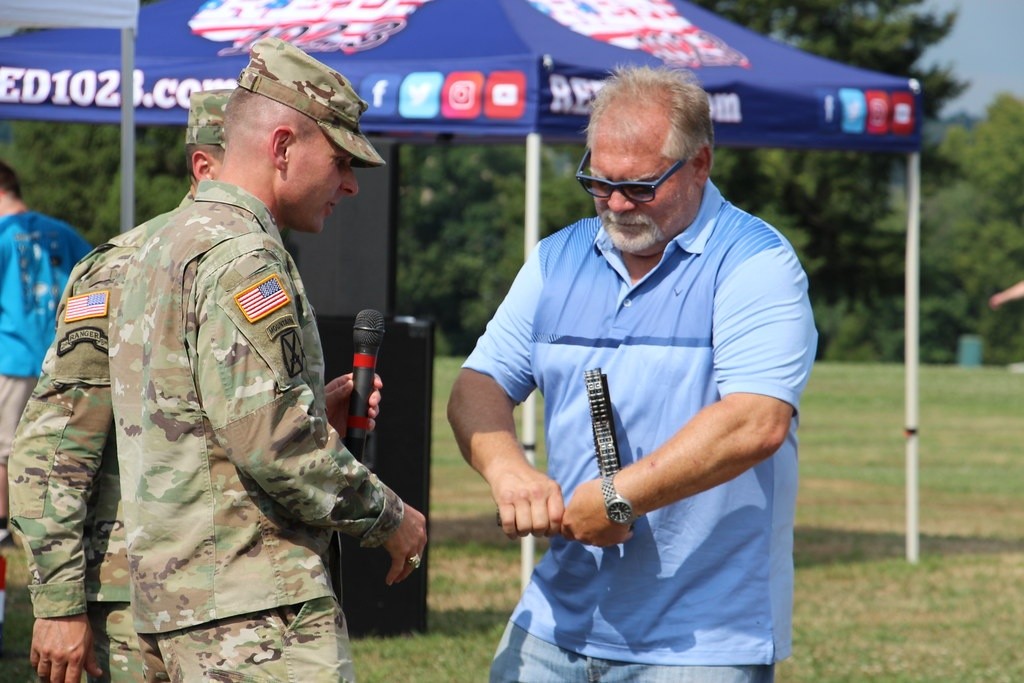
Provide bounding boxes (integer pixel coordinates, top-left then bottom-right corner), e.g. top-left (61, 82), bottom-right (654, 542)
top-left (0, 518), bottom-right (10, 543)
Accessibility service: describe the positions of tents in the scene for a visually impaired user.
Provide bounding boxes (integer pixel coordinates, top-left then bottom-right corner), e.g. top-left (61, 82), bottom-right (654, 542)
top-left (1, 1), bottom-right (923, 593)
top-left (1, 0), bottom-right (138, 234)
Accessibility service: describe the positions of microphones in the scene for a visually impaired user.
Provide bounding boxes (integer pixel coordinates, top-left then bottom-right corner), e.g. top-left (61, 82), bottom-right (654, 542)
top-left (344, 308), bottom-right (386, 464)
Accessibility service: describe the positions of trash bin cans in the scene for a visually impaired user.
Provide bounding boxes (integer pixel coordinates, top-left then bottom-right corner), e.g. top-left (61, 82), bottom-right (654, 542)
top-left (954, 333), bottom-right (986, 369)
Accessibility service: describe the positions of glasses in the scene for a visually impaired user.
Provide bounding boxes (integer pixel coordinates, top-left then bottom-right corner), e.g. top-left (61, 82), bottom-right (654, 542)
top-left (574, 147), bottom-right (684, 201)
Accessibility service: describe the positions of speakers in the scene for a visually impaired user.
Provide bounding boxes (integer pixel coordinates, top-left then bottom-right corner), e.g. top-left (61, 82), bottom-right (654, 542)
top-left (315, 314), bottom-right (437, 641)
top-left (297, 135), bottom-right (402, 319)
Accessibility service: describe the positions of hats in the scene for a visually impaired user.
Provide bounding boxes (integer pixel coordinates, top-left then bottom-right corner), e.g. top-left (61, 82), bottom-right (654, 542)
top-left (184, 90), bottom-right (234, 152)
top-left (238, 36), bottom-right (386, 169)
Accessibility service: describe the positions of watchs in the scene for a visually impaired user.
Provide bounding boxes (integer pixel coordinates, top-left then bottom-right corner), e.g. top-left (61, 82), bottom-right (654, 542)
top-left (603, 474), bottom-right (635, 526)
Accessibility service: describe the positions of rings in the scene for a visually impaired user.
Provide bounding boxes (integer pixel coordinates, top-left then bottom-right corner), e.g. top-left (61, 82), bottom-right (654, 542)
top-left (408, 555), bottom-right (421, 568)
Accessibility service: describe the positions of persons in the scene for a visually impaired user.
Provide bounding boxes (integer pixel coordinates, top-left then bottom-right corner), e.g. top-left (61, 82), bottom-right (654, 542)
top-left (1, 163), bottom-right (95, 548)
top-left (7, 88), bottom-right (237, 682)
top-left (446, 72), bottom-right (820, 683)
top-left (108, 40), bottom-right (427, 683)
